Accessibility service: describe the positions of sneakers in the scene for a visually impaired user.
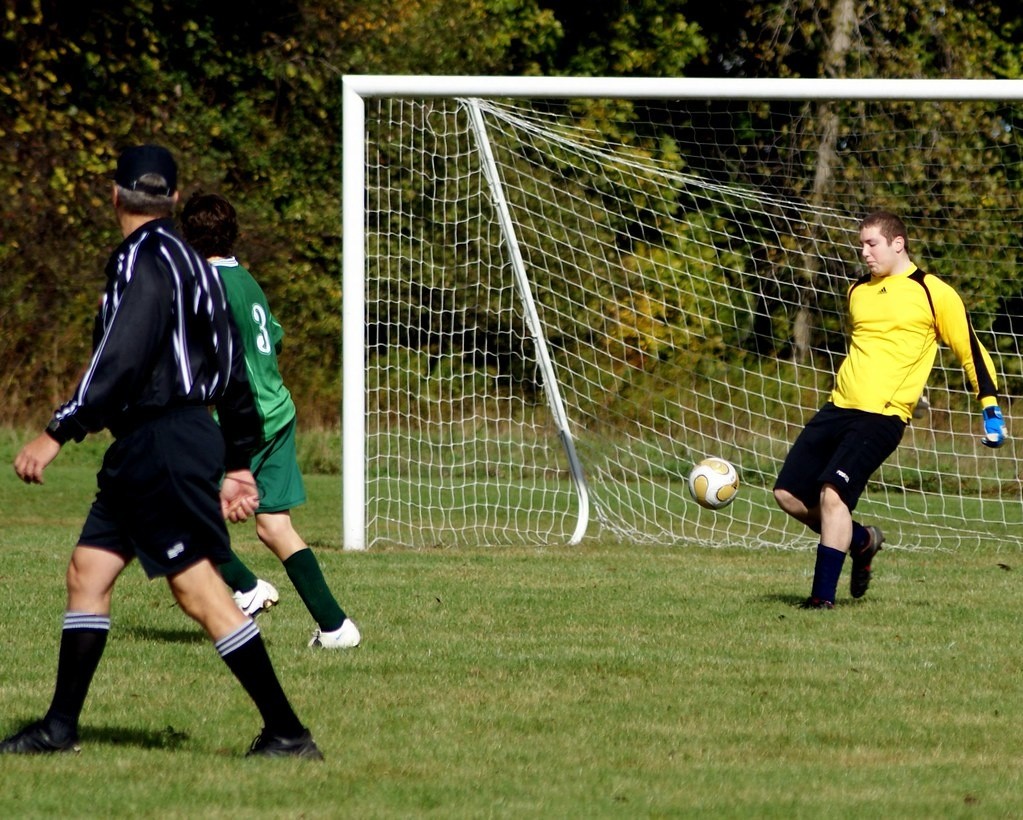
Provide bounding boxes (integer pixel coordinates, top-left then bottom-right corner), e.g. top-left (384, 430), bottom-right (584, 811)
top-left (234, 579), bottom-right (280, 617)
top-left (307, 617), bottom-right (360, 649)
top-left (797, 597), bottom-right (835, 609)
top-left (0, 718), bottom-right (82, 755)
top-left (246, 729), bottom-right (323, 761)
top-left (849, 525), bottom-right (885, 597)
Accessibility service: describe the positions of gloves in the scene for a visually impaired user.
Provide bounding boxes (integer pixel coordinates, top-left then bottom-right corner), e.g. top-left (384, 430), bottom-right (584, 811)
top-left (980, 406), bottom-right (1008, 448)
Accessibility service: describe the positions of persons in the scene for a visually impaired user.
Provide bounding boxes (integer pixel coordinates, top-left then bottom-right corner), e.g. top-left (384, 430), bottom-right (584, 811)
top-left (773, 210), bottom-right (1008, 609)
top-left (4, 144), bottom-right (323, 756)
top-left (179, 193), bottom-right (364, 652)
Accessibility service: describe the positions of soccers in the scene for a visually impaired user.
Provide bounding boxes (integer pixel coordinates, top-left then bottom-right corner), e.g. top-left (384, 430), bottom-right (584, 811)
top-left (687, 456), bottom-right (741, 511)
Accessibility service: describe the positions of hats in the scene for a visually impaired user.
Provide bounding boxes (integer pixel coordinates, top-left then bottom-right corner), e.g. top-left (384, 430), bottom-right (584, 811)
top-left (115, 144), bottom-right (178, 196)
